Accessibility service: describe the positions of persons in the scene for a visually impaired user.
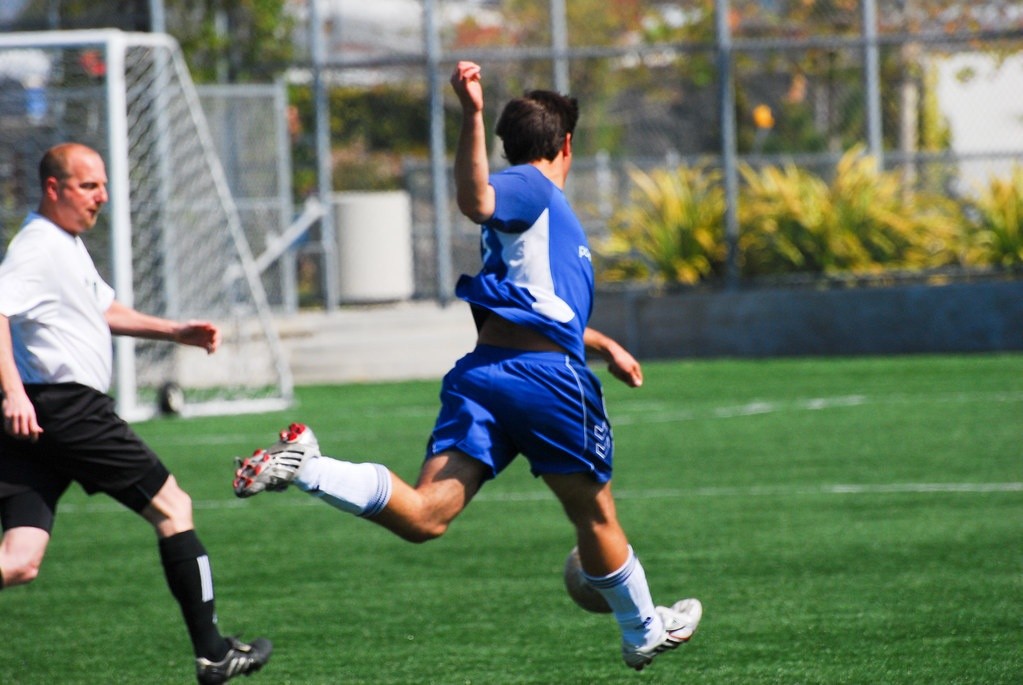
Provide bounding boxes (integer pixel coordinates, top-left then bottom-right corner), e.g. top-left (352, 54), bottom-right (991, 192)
top-left (0, 144), bottom-right (274, 685)
top-left (234, 61), bottom-right (702, 673)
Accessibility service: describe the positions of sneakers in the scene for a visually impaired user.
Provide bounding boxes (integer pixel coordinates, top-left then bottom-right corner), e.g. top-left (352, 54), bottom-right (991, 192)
top-left (195, 637), bottom-right (273, 685)
top-left (622, 598), bottom-right (702, 671)
top-left (233, 422), bottom-right (321, 498)
